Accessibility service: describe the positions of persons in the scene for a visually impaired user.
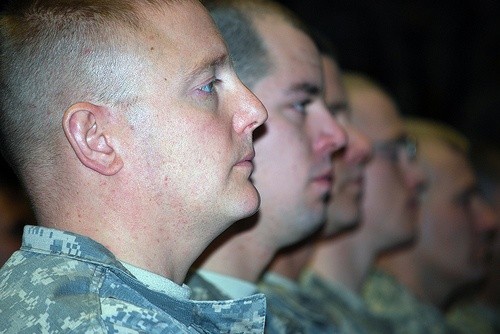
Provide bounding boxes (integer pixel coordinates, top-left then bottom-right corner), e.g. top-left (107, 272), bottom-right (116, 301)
top-left (359, 123), bottom-right (500, 333)
top-left (289, 75), bottom-right (425, 334)
top-left (178, 1), bottom-right (348, 334)
top-left (0, 0), bottom-right (267, 333)
top-left (251, 53), bottom-right (373, 333)
